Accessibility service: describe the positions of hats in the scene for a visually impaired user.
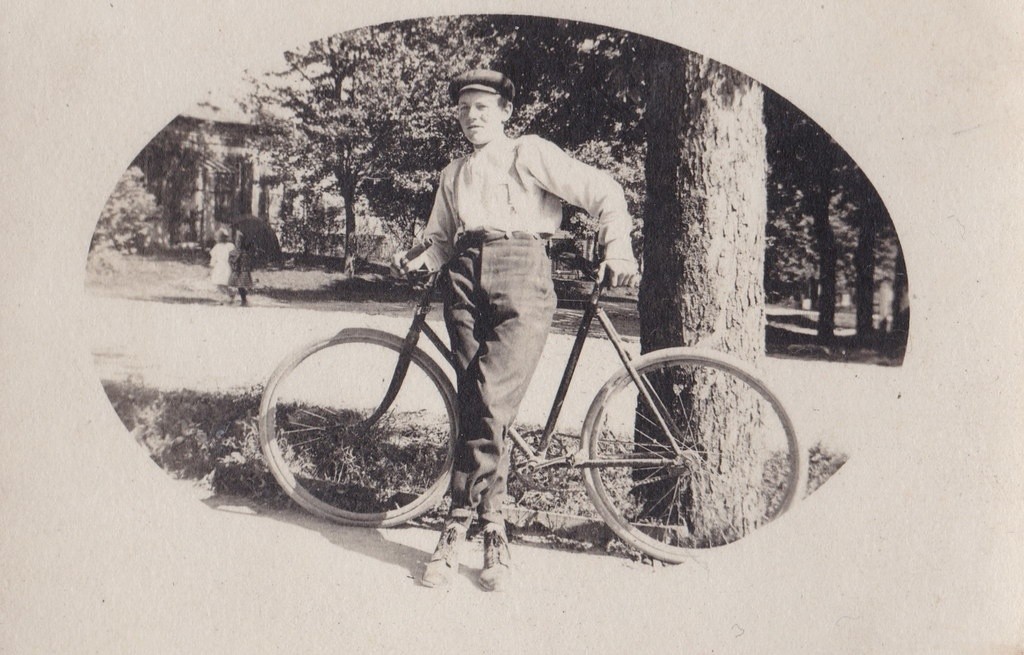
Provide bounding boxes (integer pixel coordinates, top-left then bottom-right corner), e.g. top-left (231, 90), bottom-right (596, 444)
top-left (448, 70), bottom-right (515, 103)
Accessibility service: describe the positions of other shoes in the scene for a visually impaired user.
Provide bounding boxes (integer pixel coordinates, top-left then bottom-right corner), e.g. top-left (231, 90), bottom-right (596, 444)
top-left (424, 521), bottom-right (468, 587)
top-left (478, 524), bottom-right (512, 590)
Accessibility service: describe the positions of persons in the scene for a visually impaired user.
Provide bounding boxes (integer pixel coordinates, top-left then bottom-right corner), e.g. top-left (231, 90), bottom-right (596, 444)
top-left (227, 234), bottom-right (254, 307)
top-left (204, 228), bottom-right (236, 307)
top-left (389, 70), bottom-right (643, 593)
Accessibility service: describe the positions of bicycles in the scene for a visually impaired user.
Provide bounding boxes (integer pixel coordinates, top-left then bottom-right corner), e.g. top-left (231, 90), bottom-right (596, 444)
top-left (257, 236), bottom-right (808, 565)
